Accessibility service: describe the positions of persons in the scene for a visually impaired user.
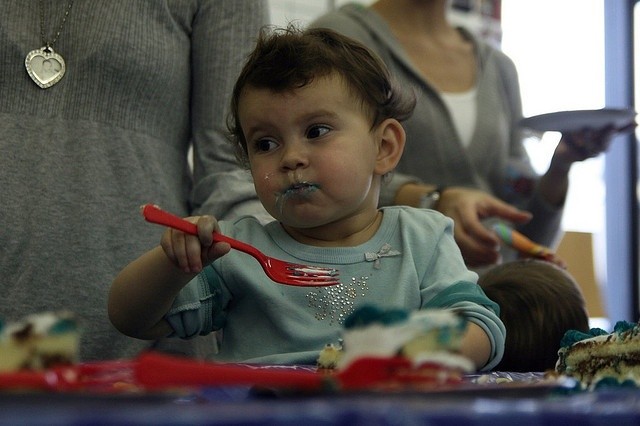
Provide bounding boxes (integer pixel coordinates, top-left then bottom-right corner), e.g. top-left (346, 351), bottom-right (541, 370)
top-left (311, 1), bottom-right (639, 264)
top-left (41, 59), bottom-right (56, 79)
top-left (461, 261), bottom-right (597, 373)
top-left (106, 27), bottom-right (508, 387)
top-left (0, 0), bottom-right (277, 387)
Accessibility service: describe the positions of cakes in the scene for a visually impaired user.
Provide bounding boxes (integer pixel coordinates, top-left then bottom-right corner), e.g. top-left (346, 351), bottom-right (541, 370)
top-left (0, 308), bottom-right (86, 373)
top-left (318, 303), bottom-right (474, 381)
top-left (556, 320), bottom-right (640, 392)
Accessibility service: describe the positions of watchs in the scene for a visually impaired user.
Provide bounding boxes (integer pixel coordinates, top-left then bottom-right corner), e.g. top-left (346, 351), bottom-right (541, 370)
top-left (418, 182), bottom-right (449, 213)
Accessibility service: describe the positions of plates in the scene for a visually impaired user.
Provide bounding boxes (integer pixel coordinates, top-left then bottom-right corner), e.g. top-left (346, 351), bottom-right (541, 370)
top-left (519, 109), bottom-right (636, 134)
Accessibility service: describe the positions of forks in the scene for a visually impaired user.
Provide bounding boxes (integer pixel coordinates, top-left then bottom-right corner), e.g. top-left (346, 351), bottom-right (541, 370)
top-left (142, 203), bottom-right (340, 288)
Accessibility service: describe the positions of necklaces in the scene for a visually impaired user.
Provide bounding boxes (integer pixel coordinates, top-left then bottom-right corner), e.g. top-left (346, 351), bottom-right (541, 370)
top-left (21, 14), bottom-right (103, 93)
